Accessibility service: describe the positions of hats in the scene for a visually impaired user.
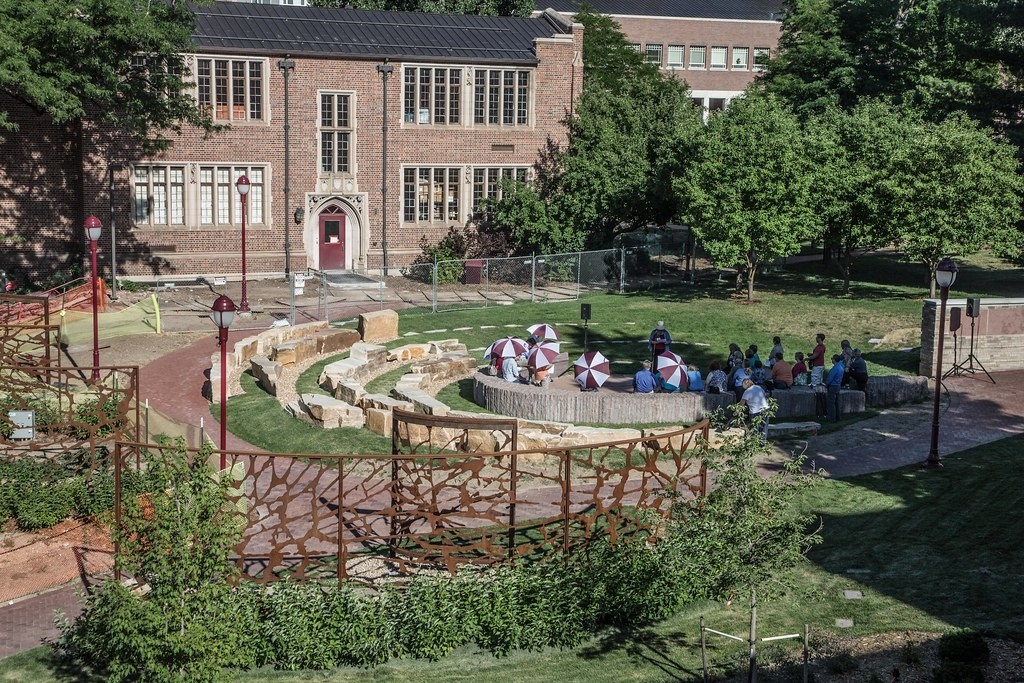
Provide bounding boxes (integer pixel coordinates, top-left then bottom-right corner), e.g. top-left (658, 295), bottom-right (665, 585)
top-left (654, 320), bottom-right (665, 329)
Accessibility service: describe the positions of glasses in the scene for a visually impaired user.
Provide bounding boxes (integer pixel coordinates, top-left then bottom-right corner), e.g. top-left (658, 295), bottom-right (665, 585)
top-left (831, 358), bottom-right (834, 360)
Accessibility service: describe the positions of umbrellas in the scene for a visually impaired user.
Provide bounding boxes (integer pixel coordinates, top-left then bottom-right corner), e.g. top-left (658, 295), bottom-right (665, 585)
top-left (526, 323), bottom-right (561, 340)
top-left (491, 335), bottom-right (529, 358)
top-left (657, 349), bottom-right (688, 388)
top-left (573, 350), bottom-right (610, 388)
top-left (484, 342), bottom-right (499, 359)
top-left (526, 339), bottom-right (560, 368)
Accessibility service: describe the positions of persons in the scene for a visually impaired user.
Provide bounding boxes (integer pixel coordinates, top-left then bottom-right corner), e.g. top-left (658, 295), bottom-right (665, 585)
top-left (489, 320), bottom-right (867, 447)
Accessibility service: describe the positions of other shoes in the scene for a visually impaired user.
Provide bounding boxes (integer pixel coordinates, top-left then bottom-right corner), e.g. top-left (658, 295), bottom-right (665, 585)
top-left (826, 417), bottom-right (842, 423)
top-left (527, 378), bottom-right (534, 383)
top-left (840, 384), bottom-right (850, 390)
top-left (533, 380), bottom-right (541, 387)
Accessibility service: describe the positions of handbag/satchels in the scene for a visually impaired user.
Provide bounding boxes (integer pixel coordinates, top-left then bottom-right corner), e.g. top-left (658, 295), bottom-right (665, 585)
top-left (793, 372), bottom-right (808, 386)
top-left (488, 363), bottom-right (497, 375)
top-left (756, 380), bottom-right (791, 392)
top-left (707, 386), bottom-right (720, 394)
